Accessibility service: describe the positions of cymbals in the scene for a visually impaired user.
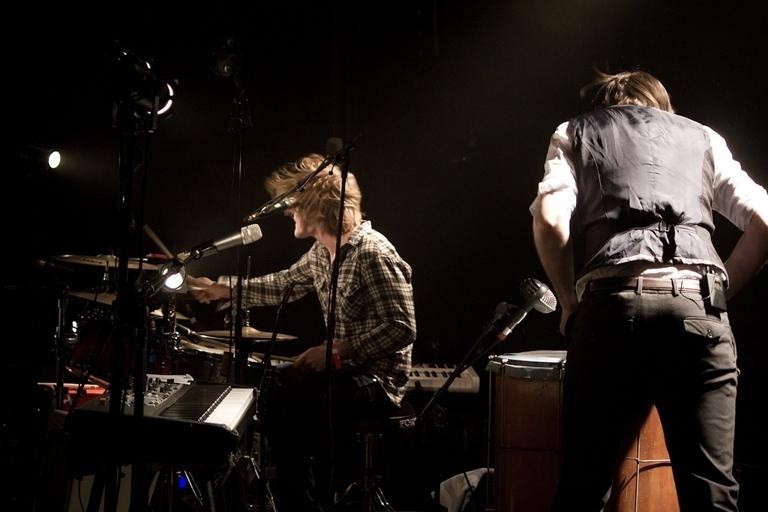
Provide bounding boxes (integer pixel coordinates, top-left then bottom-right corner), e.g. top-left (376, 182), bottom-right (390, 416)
top-left (51, 254), bottom-right (158, 272)
top-left (73, 293), bottom-right (189, 321)
top-left (203, 328), bottom-right (296, 343)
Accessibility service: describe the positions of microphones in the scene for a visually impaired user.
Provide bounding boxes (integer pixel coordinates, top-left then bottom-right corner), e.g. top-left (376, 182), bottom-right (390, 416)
top-left (177, 224), bottom-right (263, 265)
top-left (156, 317), bottom-right (202, 344)
top-left (487, 278), bottom-right (557, 342)
top-left (244, 197), bottom-right (298, 222)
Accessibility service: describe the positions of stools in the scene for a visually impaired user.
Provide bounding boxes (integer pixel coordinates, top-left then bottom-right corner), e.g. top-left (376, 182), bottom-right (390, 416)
top-left (319, 401), bottom-right (416, 512)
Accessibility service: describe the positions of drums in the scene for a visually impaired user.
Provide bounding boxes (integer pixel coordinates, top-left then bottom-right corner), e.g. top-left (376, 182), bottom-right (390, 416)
top-left (174, 339), bottom-right (269, 384)
top-left (488, 350), bottom-right (680, 511)
top-left (61, 312), bottom-right (179, 388)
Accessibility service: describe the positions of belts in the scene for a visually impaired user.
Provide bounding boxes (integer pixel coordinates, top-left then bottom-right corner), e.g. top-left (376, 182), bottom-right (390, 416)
top-left (585, 278), bottom-right (700, 291)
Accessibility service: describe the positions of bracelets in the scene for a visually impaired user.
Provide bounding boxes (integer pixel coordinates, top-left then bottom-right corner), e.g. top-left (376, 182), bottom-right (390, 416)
top-left (330, 341), bottom-right (341, 371)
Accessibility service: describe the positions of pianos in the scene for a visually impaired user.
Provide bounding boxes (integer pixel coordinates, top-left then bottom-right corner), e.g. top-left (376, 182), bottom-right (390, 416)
top-left (406, 362), bottom-right (480, 392)
top-left (74, 374), bottom-right (258, 453)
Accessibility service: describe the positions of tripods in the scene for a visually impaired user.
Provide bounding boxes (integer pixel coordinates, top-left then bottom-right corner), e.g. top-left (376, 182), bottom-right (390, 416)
top-left (196, 78), bottom-right (304, 512)
top-left (148, 333), bottom-right (206, 512)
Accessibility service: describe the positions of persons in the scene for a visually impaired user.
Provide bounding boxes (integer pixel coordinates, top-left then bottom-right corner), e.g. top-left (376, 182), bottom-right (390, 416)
top-left (188, 155), bottom-right (415, 511)
top-left (529, 68), bottom-right (768, 511)
